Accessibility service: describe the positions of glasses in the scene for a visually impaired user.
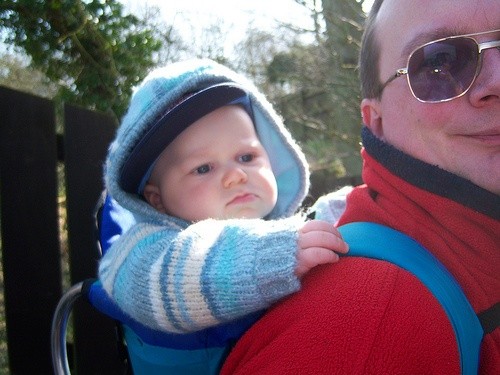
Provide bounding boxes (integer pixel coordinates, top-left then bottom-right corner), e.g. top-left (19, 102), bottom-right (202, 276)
top-left (377, 29), bottom-right (500, 104)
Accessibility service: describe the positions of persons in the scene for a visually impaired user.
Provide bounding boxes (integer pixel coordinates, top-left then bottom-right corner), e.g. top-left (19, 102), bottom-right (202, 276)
top-left (100, 58), bottom-right (355, 375)
top-left (220, 0), bottom-right (500, 375)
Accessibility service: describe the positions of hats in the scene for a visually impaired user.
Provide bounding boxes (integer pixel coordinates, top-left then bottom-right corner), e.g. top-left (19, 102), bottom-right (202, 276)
top-left (118, 82), bottom-right (254, 190)
